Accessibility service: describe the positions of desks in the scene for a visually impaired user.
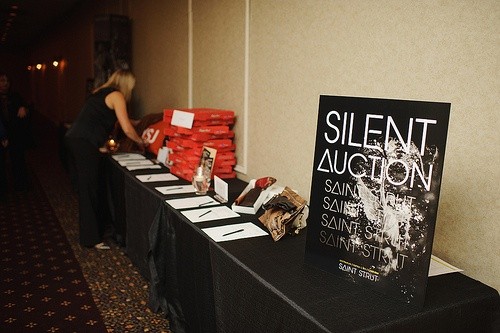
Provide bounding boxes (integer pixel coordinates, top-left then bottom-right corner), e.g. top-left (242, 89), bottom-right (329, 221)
top-left (61, 123), bottom-right (500, 333)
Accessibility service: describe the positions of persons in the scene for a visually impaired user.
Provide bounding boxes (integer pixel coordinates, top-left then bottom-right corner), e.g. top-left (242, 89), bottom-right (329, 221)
top-left (0, 73), bottom-right (32, 187)
top-left (64, 68), bottom-right (143, 248)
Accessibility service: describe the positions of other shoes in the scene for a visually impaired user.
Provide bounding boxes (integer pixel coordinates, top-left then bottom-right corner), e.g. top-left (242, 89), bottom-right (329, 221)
top-left (94, 241), bottom-right (111, 250)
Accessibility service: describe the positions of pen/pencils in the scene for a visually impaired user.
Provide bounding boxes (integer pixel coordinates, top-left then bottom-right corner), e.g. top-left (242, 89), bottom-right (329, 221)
top-left (222, 229), bottom-right (244, 236)
top-left (167, 187), bottom-right (183, 191)
top-left (198, 210), bottom-right (212, 218)
top-left (199, 201), bottom-right (214, 207)
